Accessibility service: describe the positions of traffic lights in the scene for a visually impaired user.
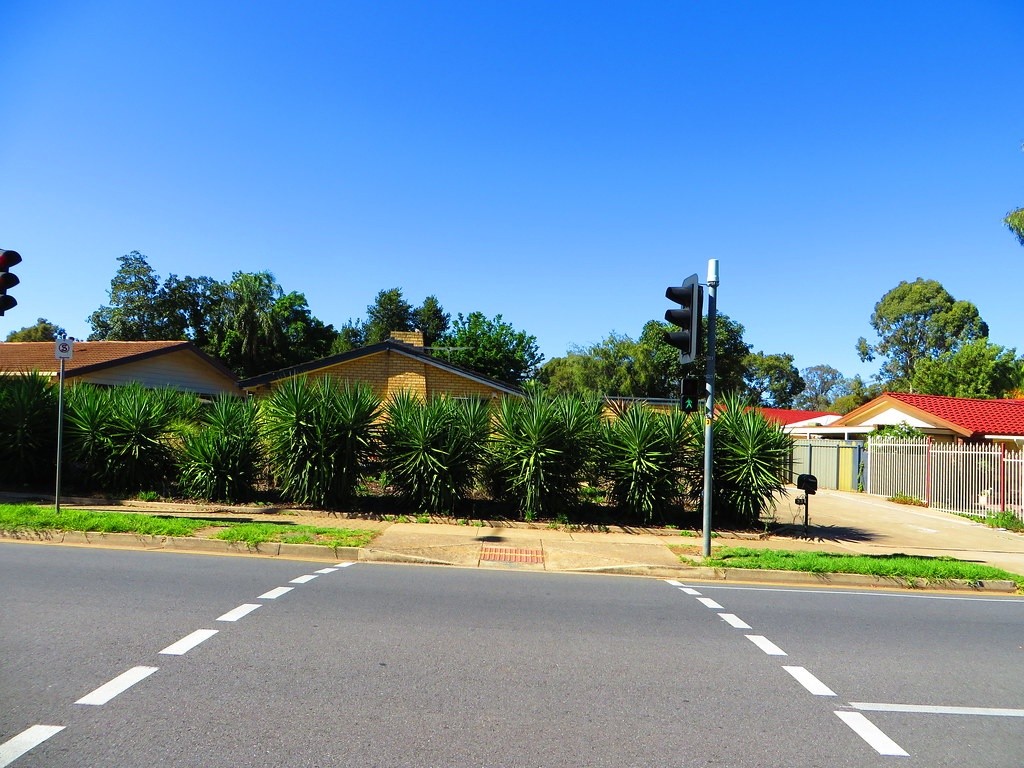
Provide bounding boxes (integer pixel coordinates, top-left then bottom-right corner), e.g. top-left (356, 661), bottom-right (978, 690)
top-left (679, 377), bottom-right (699, 413)
top-left (664, 273), bottom-right (699, 365)
top-left (0, 249), bottom-right (22, 317)
